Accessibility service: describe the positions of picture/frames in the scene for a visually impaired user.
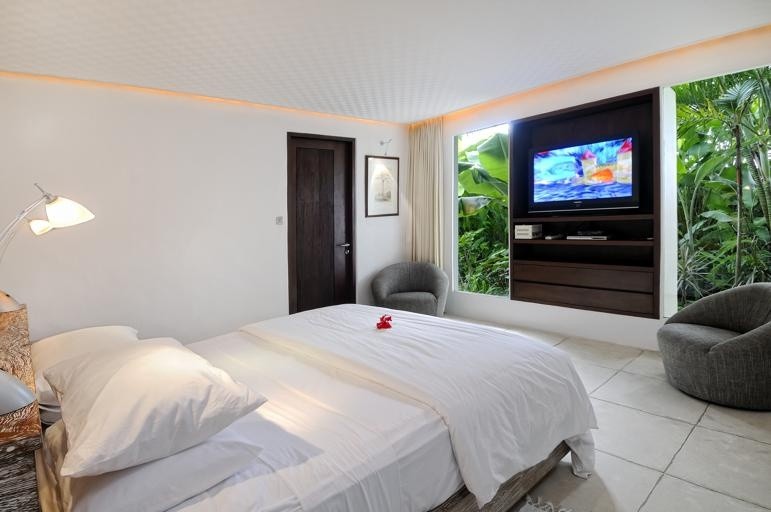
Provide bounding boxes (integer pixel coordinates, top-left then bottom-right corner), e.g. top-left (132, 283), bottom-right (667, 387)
top-left (364, 155), bottom-right (400, 218)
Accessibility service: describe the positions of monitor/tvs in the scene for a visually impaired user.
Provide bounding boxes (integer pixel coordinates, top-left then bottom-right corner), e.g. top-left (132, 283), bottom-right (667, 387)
top-left (525, 132), bottom-right (642, 214)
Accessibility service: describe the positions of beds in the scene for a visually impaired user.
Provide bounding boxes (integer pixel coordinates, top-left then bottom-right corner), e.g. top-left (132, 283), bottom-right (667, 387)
top-left (0, 290), bottom-right (599, 512)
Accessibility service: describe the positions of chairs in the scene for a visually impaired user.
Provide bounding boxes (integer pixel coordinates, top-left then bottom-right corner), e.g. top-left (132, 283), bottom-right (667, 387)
top-left (654, 282), bottom-right (770, 414)
top-left (372, 260), bottom-right (450, 318)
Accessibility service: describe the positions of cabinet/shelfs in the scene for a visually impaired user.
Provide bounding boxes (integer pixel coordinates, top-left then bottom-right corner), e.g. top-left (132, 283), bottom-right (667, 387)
top-left (509, 87), bottom-right (661, 323)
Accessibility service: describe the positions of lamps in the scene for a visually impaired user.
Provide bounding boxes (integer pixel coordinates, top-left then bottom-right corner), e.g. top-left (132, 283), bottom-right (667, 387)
top-left (0, 182), bottom-right (98, 282)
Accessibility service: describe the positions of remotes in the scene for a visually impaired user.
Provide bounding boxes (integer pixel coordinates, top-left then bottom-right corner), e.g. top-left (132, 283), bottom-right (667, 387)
top-left (545, 234), bottom-right (562, 239)
